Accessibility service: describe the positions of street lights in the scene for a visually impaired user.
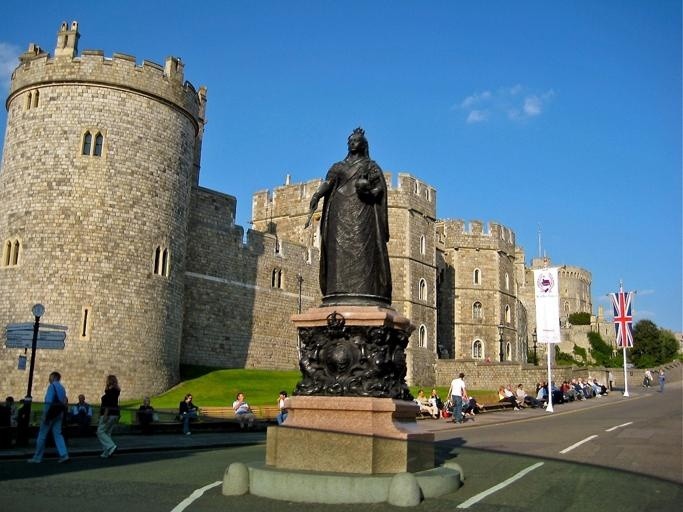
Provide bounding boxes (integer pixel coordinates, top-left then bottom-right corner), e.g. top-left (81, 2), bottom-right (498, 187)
top-left (16, 304), bottom-right (45, 447)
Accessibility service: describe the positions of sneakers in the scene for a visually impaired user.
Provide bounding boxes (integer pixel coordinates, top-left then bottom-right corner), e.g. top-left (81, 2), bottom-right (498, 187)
top-left (28, 458), bottom-right (41, 463)
top-left (101, 445), bottom-right (117, 458)
top-left (59, 456), bottom-right (69, 463)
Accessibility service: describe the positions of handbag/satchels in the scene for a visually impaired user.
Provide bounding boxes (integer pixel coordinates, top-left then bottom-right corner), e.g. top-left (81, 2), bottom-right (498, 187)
top-left (45, 394), bottom-right (64, 423)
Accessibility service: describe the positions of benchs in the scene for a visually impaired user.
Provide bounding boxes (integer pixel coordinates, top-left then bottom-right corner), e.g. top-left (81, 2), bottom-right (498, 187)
top-left (419, 380), bottom-right (611, 415)
top-left (0, 404), bottom-right (286, 436)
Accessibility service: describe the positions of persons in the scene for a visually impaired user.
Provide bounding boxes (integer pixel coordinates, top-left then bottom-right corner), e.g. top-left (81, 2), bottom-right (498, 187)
top-left (606, 371), bottom-right (613, 391)
top-left (16, 399), bottom-right (33, 425)
top-left (71, 395), bottom-right (93, 428)
top-left (642, 368), bottom-right (665, 392)
top-left (308, 127), bottom-right (393, 309)
top-left (178, 394), bottom-right (198, 436)
top-left (137, 396), bottom-right (154, 423)
top-left (276, 391), bottom-right (290, 424)
top-left (232, 393), bottom-right (255, 432)
top-left (95, 374), bottom-right (121, 458)
top-left (416, 373), bottom-right (483, 425)
top-left (498, 378), bottom-right (607, 410)
top-left (27, 372), bottom-right (70, 464)
top-left (3, 395), bottom-right (19, 444)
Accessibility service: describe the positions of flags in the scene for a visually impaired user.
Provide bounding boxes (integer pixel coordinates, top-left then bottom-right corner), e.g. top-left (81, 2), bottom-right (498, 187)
top-left (611, 292), bottom-right (634, 349)
